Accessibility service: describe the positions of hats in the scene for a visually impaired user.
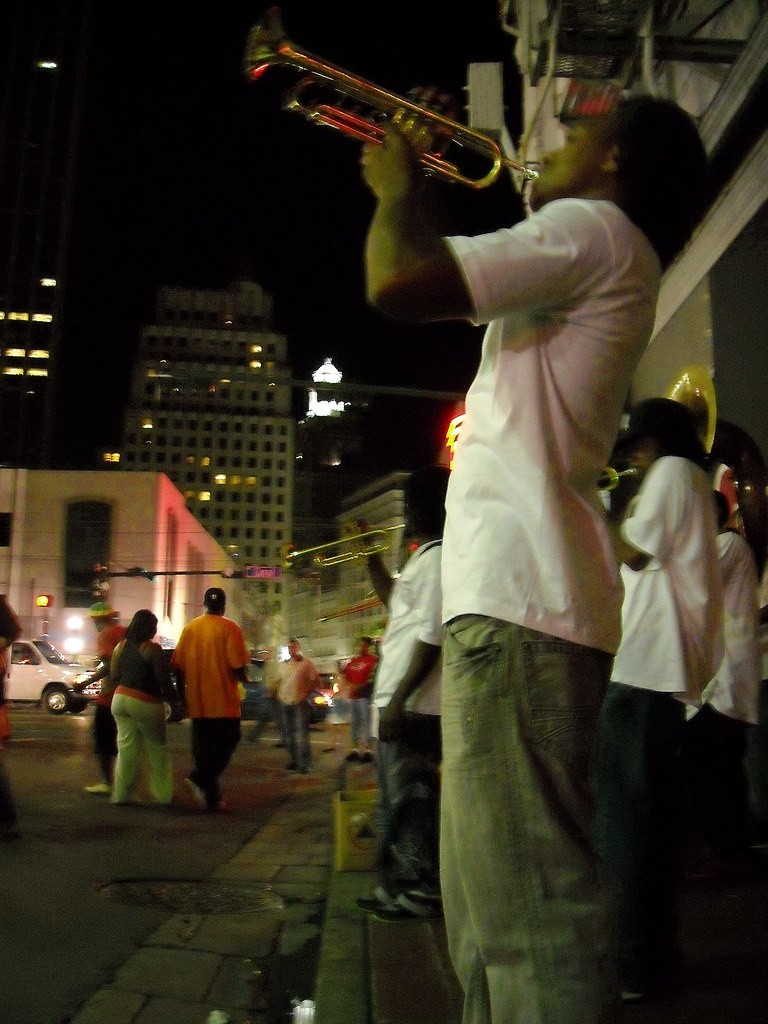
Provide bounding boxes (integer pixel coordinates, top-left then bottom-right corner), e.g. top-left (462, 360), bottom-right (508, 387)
top-left (615, 398), bottom-right (694, 451)
top-left (202, 588), bottom-right (225, 606)
top-left (89, 602), bottom-right (118, 619)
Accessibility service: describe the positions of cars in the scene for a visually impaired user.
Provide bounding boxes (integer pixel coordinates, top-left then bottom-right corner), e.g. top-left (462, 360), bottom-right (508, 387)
top-left (159, 646), bottom-right (332, 729)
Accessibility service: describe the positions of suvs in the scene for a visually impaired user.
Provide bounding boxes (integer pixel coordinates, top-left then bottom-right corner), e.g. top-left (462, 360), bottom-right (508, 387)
top-left (5, 638), bottom-right (103, 716)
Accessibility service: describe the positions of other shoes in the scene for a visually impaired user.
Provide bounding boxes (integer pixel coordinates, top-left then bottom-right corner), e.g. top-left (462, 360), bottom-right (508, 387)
top-left (362, 752), bottom-right (375, 762)
top-left (286, 762), bottom-right (300, 771)
top-left (372, 900), bottom-right (443, 924)
top-left (618, 985), bottom-right (661, 1005)
top-left (356, 896), bottom-right (384, 912)
top-left (185, 777), bottom-right (208, 811)
top-left (82, 782), bottom-right (113, 793)
top-left (211, 799), bottom-right (227, 810)
top-left (347, 752), bottom-right (362, 763)
top-left (299, 774), bottom-right (311, 788)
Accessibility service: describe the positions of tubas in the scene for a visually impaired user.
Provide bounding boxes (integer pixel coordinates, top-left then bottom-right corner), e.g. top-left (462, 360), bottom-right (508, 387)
top-left (661, 362), bottom-right (767, 587)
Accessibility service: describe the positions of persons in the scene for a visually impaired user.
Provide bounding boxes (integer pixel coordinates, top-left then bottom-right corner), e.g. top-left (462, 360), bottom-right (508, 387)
top-left (73, 587), bottom-right (321, 807)
top-left (333, 396), bottom-right (768, 1008)
top-left (0, 595), bottom-right (23, 839)
top-left (360, 94), bottom-right (713, 1024)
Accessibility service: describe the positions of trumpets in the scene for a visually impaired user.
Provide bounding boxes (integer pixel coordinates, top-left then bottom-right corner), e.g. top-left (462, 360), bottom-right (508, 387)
top-left (280, 521), bottom-right (408, 572)
top-left (241, 2), bottom-right (541, 192)
top-left (316, 594), bottom-right (384, 626)
top-left (596, 465), bottom-right (639, 493)
